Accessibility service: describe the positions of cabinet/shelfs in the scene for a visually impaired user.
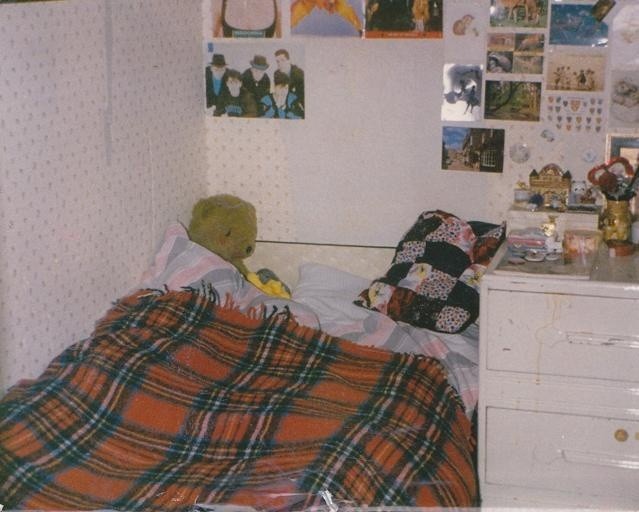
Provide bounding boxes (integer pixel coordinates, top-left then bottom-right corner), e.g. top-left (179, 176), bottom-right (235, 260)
top-left (461, 226), bottom-right (639, 509)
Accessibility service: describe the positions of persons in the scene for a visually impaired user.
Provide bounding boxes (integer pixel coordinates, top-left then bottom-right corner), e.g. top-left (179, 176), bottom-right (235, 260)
top-left (206, 54), bottom-right (240, 109)
top-left (273, 49), bottom-right (304, 105)
top-left (214, 69), bottom-right (258, 118)
top-left (240, 55), bottom-right (271, 99)
top-left (258, 70), bottom-right (305, 119)
top-left (212, 1), bottom-right (282, 39)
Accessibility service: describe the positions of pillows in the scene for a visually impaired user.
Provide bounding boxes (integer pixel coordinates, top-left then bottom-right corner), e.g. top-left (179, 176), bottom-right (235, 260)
top-left (289, 261), bottom-right (375, 319)
top-left (350, 204), bottom-right (510, 335)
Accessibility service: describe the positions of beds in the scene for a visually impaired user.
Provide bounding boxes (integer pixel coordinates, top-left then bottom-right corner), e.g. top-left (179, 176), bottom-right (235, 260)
top-left (2, 218), bottom-right (480, 510)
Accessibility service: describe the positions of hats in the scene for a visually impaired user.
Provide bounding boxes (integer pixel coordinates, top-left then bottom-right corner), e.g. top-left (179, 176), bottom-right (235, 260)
top-left (250, 54), bottom-right (270, 70)
top-left (209, 54), bottom-right (230, 67)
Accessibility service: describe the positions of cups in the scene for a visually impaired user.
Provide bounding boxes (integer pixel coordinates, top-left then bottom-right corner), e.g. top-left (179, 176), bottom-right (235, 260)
top-left (514, 189), bottom-right (533, 208)
top-left (564, 230), bottom-right (602, 275)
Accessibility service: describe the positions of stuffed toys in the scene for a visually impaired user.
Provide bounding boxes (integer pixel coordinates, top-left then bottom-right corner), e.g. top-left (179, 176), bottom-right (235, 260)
top-left (189, 193), bottom-right (290, 300)
